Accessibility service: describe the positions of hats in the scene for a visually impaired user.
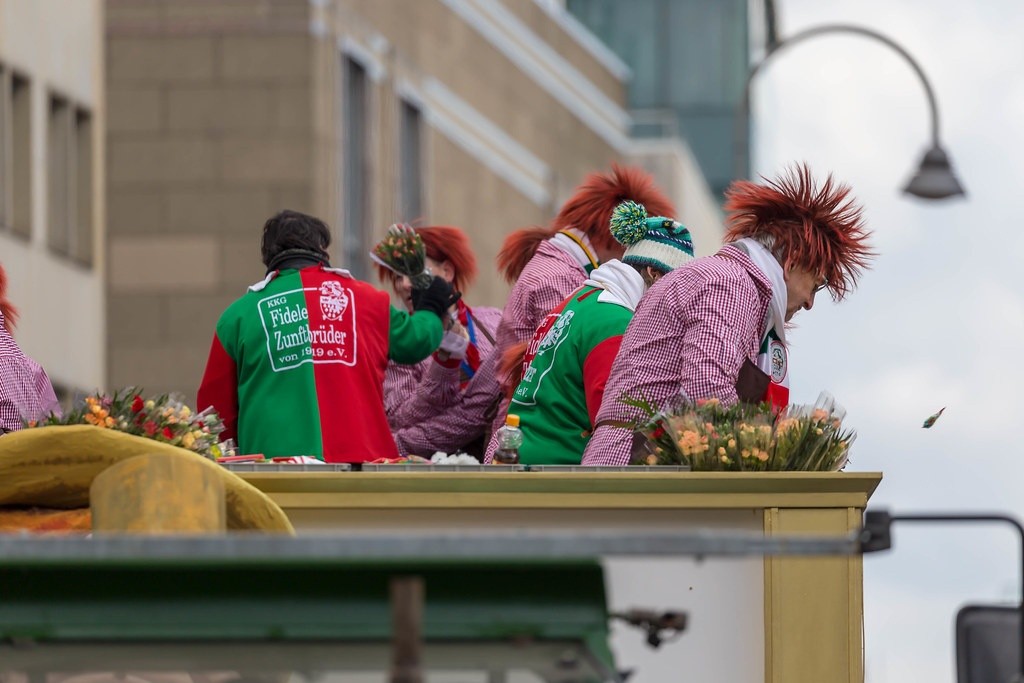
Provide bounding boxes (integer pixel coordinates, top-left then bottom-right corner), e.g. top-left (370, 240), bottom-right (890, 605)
top-left (609, 201), bottom-right (694, 275)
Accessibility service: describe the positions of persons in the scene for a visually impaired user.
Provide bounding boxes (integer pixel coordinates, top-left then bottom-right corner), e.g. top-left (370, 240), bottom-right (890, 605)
top-left (484, 200), bottom-right (695, 464)
top-left (492, 160), bottom-right (676, 442)
top-left (194, 209), bottom-right (463, 472)
top-left (0, 261), bottom-right (64, 434)
top-left (371, 214), bottom-right (506, 463)
top-left (579, 157), bottom-right (883, 465)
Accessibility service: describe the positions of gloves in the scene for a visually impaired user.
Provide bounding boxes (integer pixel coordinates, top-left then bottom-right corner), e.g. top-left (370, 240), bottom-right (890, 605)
top-left (411, 276), bottom-right (462, 316)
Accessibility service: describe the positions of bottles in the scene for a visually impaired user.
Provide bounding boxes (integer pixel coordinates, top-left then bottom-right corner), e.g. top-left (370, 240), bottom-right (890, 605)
top-left (490, 413), bottom-right (524, 466)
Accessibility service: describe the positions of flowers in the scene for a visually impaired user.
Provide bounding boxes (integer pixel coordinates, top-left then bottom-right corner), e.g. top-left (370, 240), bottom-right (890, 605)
top-left (633, 389), bottom-right (859, 476)
top-left (21, 386), bottom-right (239, 465)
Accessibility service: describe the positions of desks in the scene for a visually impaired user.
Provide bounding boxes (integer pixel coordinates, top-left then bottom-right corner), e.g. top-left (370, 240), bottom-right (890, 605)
top-left (1, 464), bottom-right (882, 683)
